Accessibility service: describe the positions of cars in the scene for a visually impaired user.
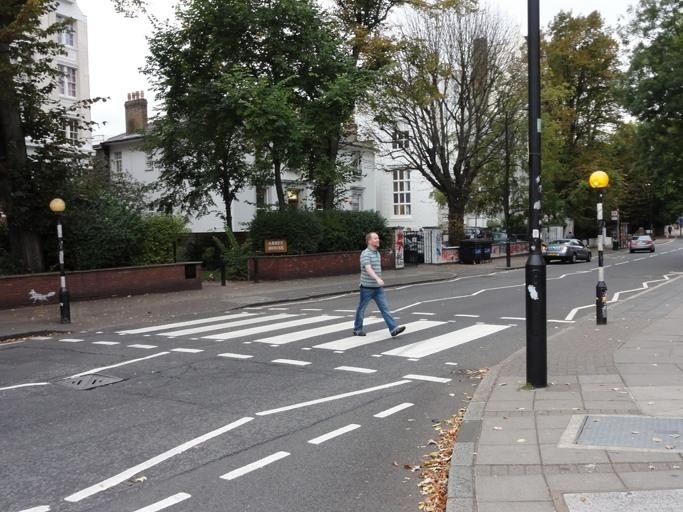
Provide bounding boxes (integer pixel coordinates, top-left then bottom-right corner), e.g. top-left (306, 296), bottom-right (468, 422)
top-left (628, 235), bottom-right (655, 253)
top-left (543, 238), bottom-right (592, 264)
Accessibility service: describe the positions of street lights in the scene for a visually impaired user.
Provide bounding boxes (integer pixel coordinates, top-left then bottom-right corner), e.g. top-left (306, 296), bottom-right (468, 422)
top-left (48, 197), bottom-right (71, 325)
top-left (590, 170), bottom-right (610, 328)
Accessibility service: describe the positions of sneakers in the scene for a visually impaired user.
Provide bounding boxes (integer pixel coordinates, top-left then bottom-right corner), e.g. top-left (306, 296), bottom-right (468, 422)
top-left (354, 328), bottom-right (365, 336)
top-left (391, 326), bottom-right (404, 336)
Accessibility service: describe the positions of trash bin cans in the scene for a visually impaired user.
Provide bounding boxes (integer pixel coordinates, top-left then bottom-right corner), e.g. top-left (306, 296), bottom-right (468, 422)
top-left (460, 239), bottom-right (492, 264)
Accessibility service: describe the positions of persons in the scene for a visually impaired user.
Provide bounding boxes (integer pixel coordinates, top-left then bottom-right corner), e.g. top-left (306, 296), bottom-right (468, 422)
top-left (354, 231), bottom-right (405, 337)
top-left (566, 232), bottom-right (575, 239)
